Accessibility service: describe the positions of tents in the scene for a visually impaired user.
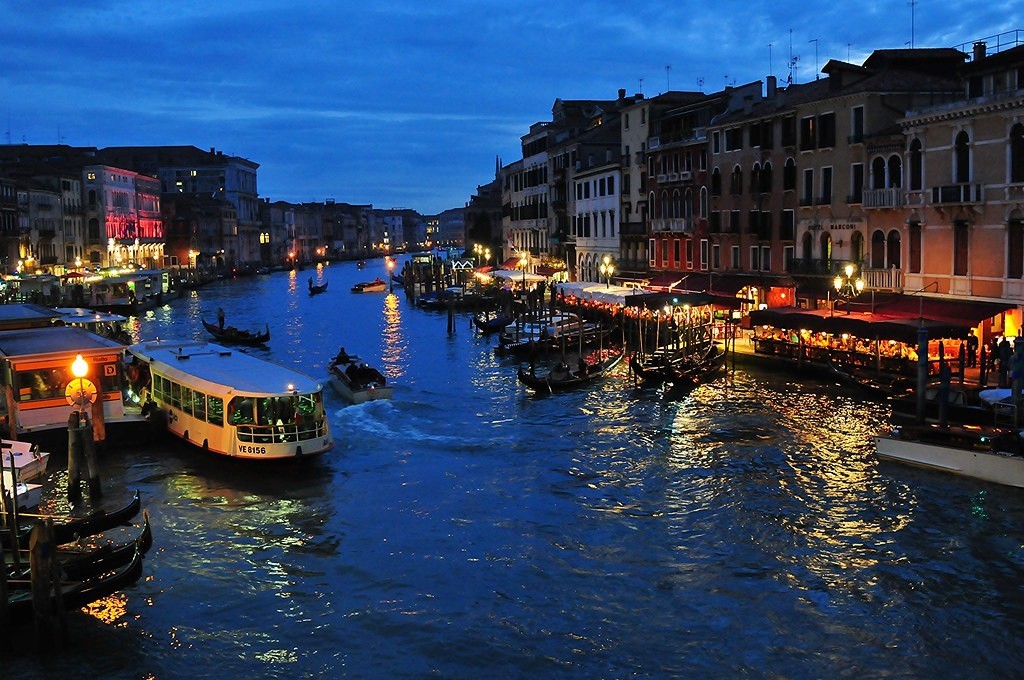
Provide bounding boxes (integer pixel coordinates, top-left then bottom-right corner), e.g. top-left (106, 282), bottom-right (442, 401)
top-left (488, 270), bottom-right (547, 289)
top-left (749, 305), bottom-right (970, 345)
top-left (557, 281), bottom-right (740, 317)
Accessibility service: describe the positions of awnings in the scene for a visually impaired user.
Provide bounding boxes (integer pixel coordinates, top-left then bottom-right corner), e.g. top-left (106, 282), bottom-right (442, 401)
top-left (537, 268), bottom-right (560, 276)
top-left (501, 257), bottom-right (521, 269)
top-left (836, 293), bottom-right (1015, 328)
top-left (642, 274), bottom-right (755, 297)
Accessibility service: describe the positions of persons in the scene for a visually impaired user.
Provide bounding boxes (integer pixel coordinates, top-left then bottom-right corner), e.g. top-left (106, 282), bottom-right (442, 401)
top-left (336, 347), bottom-right (349, 363)
top-left (755, 325), bottom-right (932, 371)
top-left (346, 362), bottom-right (358, 374)
top-left (234, 397), bottom-right (293, 440)
top-left (109, 356), bottom-right (151, 394)
top-left (31, 369), bottom-right (62, 391)
top-left (569, 295), bottom-right (711, 323)
top-left (965, 330), bottom-right (978, 368)
top-left (989, 336), bottom-right (1010, 375)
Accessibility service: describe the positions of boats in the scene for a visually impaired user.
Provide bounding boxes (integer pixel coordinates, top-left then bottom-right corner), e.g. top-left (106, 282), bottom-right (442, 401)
top-left (473, 300), bottom-right (619, 356)
top-left (351, 279), bottom-right (388, 293)
top-left (889, 379), bottom-right (1024, 442)
top-left (630, 298), bottom-right (730, 386)
top-left (872, 435), bottom-right (1024, 489)
top-left (308, 281), bottom-right (329, 295)
top-left (328, 353), bottom-right (393, 405)
top-left (0, 437), bottom-right (51, 513)
top-left (202, 317), bottom-right (270, 344)
top-left (391, 252), bottom-right (453, 290)
top-left (517, 291), bottom-right (624, 390)
top-left (125, 337), bottom-right (335, 461)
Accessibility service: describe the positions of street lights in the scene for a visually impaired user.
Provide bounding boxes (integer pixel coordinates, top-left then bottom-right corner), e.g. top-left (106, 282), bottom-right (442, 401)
top-left (832, 259), bottom-right (867, 314)
top-left (598, 253), bottom-right (616, 288)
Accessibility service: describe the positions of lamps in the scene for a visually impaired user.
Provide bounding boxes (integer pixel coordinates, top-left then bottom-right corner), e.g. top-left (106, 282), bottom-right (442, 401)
top-left (72, 354), bottom-right (88, 411)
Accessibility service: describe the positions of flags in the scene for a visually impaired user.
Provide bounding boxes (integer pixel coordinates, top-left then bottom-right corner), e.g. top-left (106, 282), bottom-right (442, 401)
top-left (506, 234), bottom-right (514, 248)
